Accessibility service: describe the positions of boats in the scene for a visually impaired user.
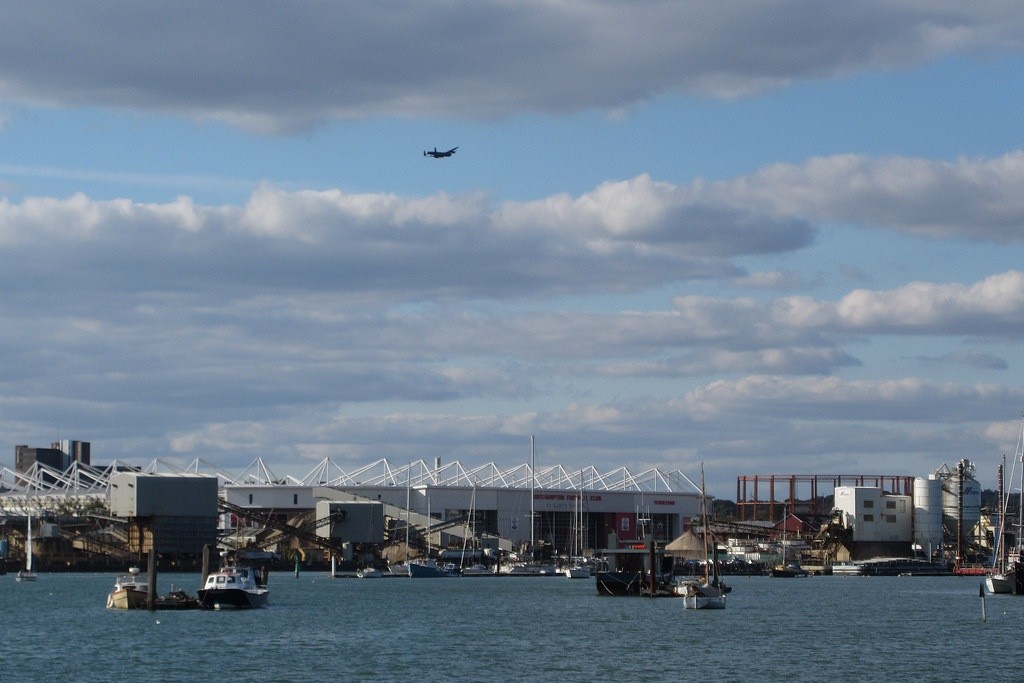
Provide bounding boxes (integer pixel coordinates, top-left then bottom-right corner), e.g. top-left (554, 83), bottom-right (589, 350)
top-left (140, 583), bottom-right (200, 612)
top-left (356, 565), bottom-right (382, 578)
top-left (106, 565), bottom-right (149, 611)
top-left (593, 570), bottom-right (676, 596)
top-left (197, 562), bottom-right (271, 611)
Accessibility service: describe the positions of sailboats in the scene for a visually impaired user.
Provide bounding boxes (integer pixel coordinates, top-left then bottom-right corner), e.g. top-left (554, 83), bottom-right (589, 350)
top-left (771, 506), bottom-right (807, 578)
top-left (498, 434), bottom-right (565, 577)
top-left (17, 510), bottom-right (38, 582)
top-left (565, 469), bottom-right (590, 580)
top-left (682, 461), bottom-right (728, 608)
top-left (395, 482), bottom-right (496, 578)
top-left (983, 415), bottom-right (1024, 595)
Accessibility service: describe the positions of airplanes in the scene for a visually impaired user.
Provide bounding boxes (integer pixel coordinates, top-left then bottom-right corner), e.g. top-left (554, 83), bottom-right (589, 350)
top-left (424, 146), bottom-right (459, 159)
